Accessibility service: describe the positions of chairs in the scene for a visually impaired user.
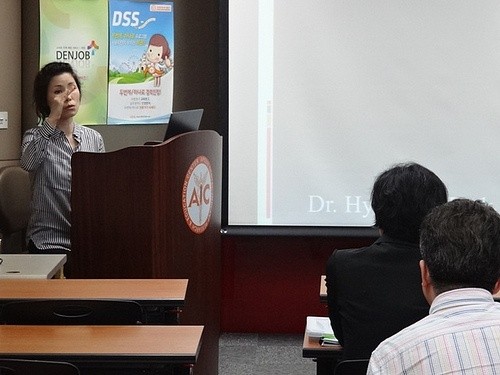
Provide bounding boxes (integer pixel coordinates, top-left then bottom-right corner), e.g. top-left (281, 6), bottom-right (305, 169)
top-left (0, 166), bottom-right (33, 252)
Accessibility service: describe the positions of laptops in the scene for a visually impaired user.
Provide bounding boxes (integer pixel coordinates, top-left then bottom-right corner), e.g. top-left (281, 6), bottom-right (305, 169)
top-left (163, 109), bottom-right (203, 142)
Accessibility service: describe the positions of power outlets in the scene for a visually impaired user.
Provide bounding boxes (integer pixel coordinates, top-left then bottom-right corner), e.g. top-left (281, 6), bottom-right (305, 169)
top-left (0, 111), bottom-right (8, 128)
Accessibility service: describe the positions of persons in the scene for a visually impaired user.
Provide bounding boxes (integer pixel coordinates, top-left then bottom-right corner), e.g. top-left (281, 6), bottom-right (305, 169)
top-left (19, 62), bottom-right (106, 279)
top-left (366, 198), bottom-right (500, 375)
top-left (324, 162), bottom-right (449, 360)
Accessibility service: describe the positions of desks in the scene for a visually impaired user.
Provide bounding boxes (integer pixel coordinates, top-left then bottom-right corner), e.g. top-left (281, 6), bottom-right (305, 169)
top-left (0, 325), bottom-right (205, 375)
top-left (0, 278), bottom-right (189, 324)
top-left (0, 254), bottom-right (68, 280)
top-left (302, 316), bottom-right (343, 375)
